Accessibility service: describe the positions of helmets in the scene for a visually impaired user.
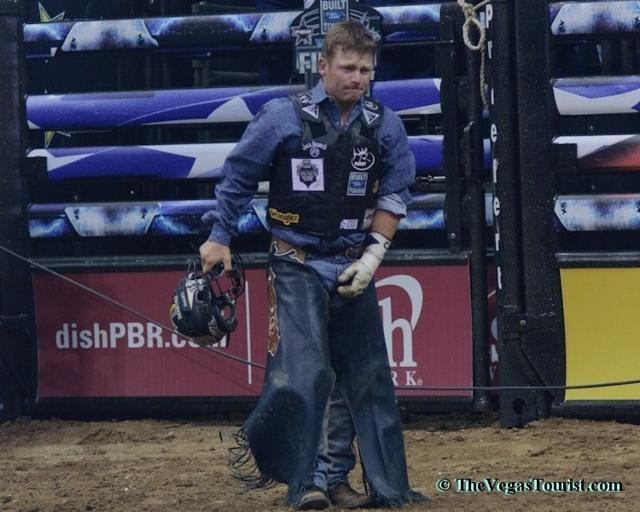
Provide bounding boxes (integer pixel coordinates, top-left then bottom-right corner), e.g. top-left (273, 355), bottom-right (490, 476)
top-left (170, 272), bottom-right (237, 345)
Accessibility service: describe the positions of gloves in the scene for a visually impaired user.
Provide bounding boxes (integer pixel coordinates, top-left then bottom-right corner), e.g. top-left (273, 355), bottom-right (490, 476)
top-left (337, 230), bottom-right (391, 297)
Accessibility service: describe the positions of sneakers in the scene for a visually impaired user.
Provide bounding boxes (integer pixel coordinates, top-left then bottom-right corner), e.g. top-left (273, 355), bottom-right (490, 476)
top-left (296, 488), bottom-right (329, 510)
top-left (325, 483), bottom-right (372, 508)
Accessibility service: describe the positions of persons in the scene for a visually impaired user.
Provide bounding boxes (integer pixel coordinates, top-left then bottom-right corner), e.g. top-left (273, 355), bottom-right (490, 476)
top-left (199, 18), bottom-right (429, 511)
